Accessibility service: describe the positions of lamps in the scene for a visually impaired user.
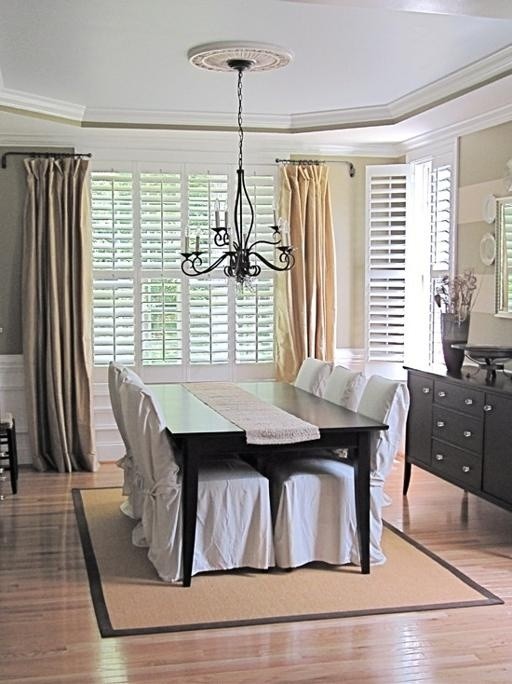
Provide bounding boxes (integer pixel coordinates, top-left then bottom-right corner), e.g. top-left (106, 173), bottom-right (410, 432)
top-left (180, 40), bottom-right (298, 280)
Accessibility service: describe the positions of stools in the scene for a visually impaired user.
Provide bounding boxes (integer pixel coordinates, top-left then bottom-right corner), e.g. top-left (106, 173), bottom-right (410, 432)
top-left (0, 411), bottom-right (18, 495)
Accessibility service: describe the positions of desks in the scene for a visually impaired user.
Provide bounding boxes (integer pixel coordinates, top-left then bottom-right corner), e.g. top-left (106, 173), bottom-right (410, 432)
top-left (143, 382), bottom-right (389, 588)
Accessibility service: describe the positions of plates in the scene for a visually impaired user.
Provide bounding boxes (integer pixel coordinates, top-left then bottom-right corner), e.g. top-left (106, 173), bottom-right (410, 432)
top-left (479, 193), bottom-right (497, 265)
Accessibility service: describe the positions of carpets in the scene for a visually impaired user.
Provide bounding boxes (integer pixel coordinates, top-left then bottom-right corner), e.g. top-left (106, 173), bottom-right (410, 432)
top-left (71, 485), bottom-right (505, 639)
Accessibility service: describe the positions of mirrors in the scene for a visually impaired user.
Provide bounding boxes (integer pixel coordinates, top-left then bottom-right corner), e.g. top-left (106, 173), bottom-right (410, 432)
top-left (494, 195), bottom-right (512, 320)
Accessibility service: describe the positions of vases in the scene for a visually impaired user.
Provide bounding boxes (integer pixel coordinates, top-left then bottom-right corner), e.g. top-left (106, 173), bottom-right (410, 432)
top-left (439, 313), bottom-right (470, 372)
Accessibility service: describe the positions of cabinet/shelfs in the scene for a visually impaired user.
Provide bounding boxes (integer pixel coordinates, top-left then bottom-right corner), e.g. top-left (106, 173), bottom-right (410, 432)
top-left (402, 363), bottom-right (512, 513)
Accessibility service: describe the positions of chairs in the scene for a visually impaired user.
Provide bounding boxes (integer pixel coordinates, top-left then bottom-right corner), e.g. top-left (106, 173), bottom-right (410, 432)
top-left (108, 359), bottom-right (275, 581)
top-left (255, 356), bottom-right (366, 467)
top-left (274, 376), bottom-right (411, 571)
